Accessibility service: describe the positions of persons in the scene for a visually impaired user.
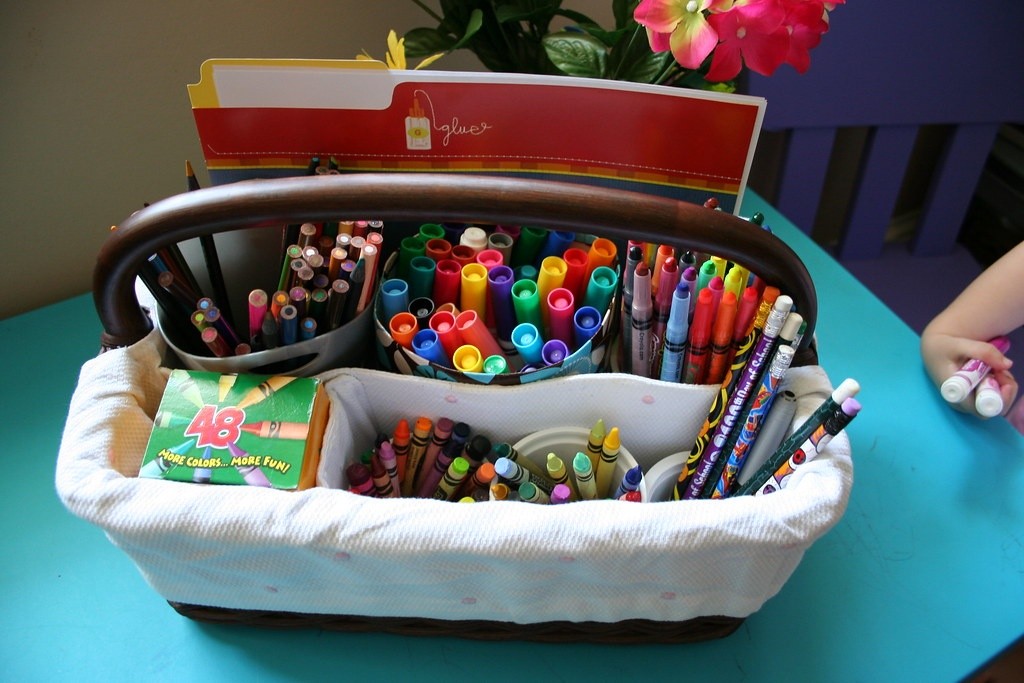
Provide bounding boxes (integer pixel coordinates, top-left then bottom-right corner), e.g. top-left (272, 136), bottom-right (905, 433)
top-left (921, 240), bottom-right (1024, 419)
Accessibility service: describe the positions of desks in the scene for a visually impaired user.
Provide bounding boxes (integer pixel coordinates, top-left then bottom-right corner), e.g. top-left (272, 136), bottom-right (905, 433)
top-left (0, 190), bottom-right (1024, 683)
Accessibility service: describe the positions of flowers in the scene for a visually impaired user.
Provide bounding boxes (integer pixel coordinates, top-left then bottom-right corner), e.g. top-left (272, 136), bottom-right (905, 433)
top-left (350, 0), bottom-right (850, 97)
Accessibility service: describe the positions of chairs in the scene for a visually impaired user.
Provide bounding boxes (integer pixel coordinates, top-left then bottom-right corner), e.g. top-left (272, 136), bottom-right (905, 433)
top-left (738, 0), bottom-right (1024, 418)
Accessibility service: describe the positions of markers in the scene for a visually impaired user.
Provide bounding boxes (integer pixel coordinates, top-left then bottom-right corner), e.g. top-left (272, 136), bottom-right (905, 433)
top-left (381, 220), bottom-right (618, 376)
top-left (940, 337), bottom-right (1013, 416)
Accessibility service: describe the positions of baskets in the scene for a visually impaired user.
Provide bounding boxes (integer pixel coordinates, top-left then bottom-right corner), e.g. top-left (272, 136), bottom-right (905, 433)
top-left (96, 170), bottom-right (818, 645)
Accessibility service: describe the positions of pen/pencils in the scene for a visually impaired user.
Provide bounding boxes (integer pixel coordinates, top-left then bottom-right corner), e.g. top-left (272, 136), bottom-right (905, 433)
top-left (109, 155), bottom-right (383, 356)
top-left (671, 293), bottom-right (859, 501)
